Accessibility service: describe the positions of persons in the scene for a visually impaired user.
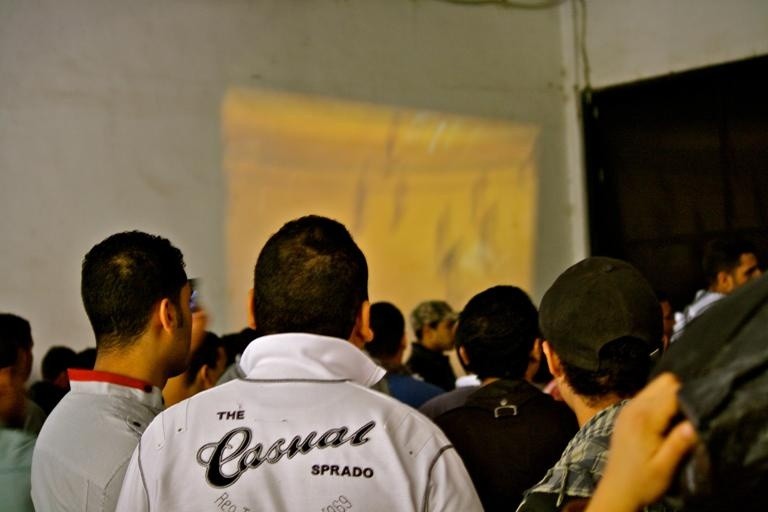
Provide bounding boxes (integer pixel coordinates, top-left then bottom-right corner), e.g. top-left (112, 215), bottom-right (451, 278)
top-left (2, 228), bottom-right (252, 509)
top-left (365, 233), bottom-right (767, 512)
top-left (116, 215), bottom-right (482, 509)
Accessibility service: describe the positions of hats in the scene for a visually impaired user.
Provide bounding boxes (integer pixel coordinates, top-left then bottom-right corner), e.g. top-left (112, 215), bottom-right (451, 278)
top-left (410, 301), bottom-right (462, 332)
top-left (538, 257), bottom-right (663, 371)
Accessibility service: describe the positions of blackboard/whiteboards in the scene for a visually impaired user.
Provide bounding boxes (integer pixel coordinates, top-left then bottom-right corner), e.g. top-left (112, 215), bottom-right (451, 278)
top-left (581, 52), bottom-right (766, 315)
